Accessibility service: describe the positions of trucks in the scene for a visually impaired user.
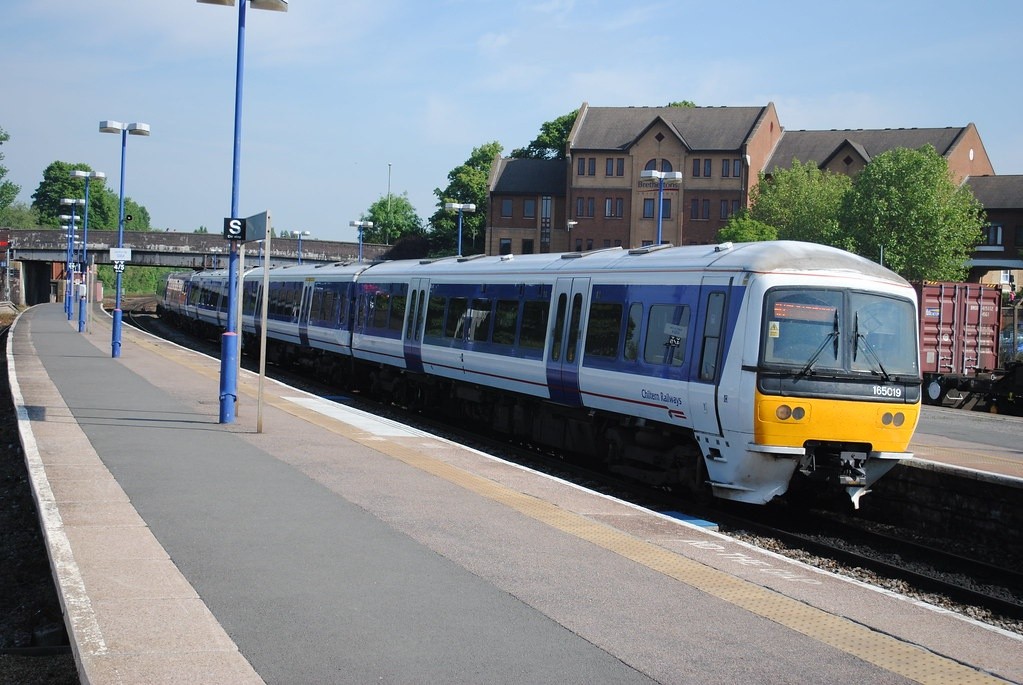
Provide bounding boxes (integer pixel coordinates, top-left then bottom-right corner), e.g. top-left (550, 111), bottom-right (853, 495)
top-left (908, 276), bottom-right (1004, 409)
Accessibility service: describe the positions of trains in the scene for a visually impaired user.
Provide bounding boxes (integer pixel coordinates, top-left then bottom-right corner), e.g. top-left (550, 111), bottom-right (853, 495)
top-left (156, 236), bottom-right (924, 520)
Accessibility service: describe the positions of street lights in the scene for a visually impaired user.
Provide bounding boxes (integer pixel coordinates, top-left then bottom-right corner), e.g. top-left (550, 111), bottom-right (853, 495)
top-left (443, 202), bottom-right (476, 255)
top-left (640, 169), bottom-right (683, 244)
top-left (191, 0), bottom-right (290, 422)
top-left (70, 168), bottom-right (106, 331)
top-left (58, 195), bottom-right (86, 319)
top-left (291, 229), bottom-right (311, 265)
top-left (349, 219), bottom-right (373, 261)
top-left (99, 120), bottom-right (153, 357)
